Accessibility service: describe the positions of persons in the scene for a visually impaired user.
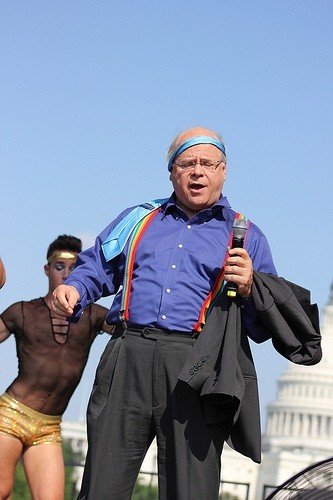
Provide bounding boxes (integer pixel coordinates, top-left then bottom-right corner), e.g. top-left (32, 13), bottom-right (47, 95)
top-left (0, 235), bottom-right (117, 500)
top-left (51, 127), bottom-right (278, 500)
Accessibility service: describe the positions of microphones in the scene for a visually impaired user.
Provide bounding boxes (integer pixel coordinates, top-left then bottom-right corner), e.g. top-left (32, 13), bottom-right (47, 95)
top-left (226, 219), bottom-right (248, 296)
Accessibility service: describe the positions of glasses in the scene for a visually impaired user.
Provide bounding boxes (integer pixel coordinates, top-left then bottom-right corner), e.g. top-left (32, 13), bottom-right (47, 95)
top-left (170, 153), bottom-right (226, 170)
